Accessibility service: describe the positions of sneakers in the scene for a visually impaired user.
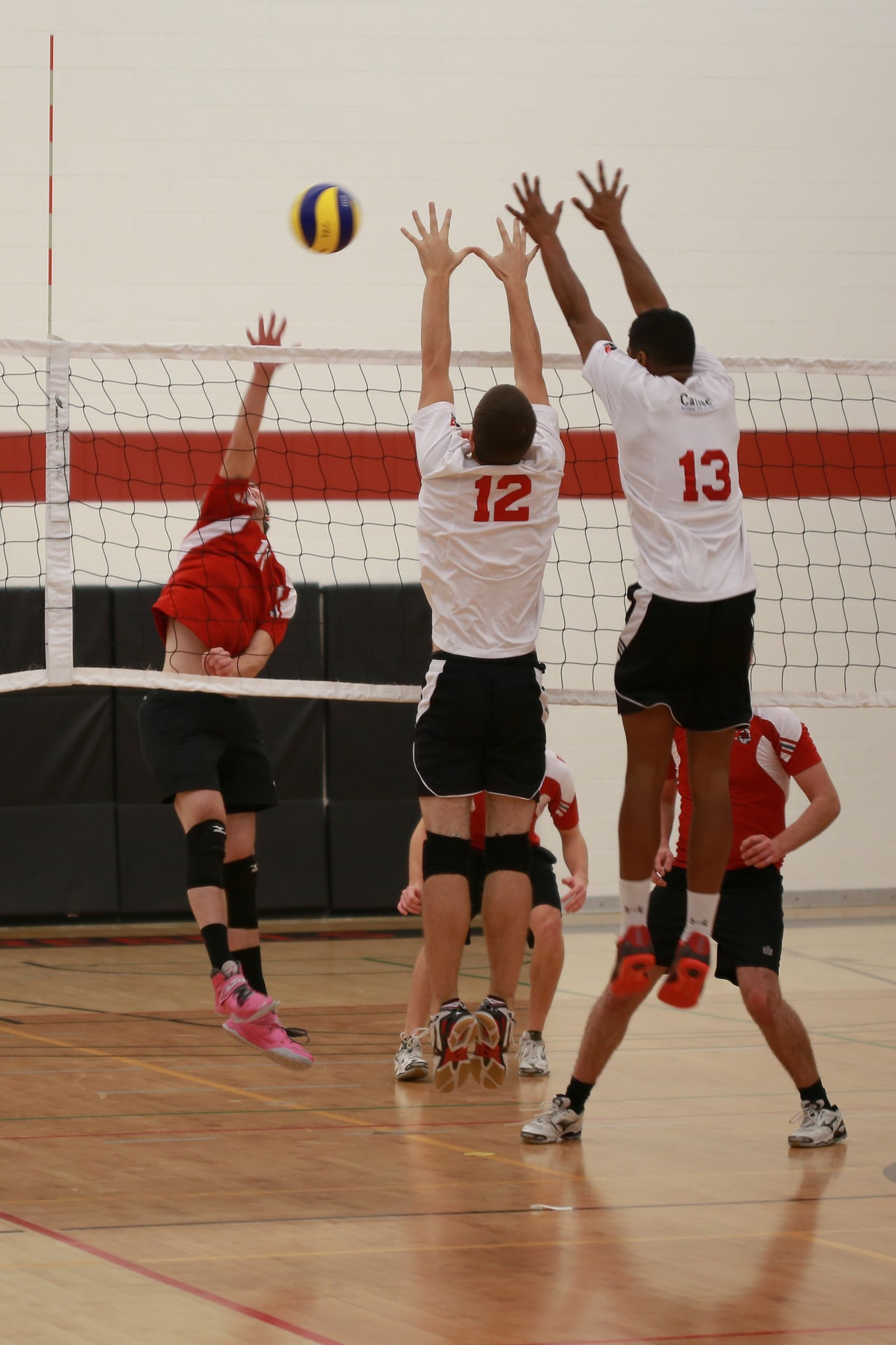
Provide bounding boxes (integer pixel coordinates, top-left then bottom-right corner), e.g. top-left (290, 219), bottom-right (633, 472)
top-left (468, 996), bottom-right (515, 1090)
top-left (394, 1027), bottom-right (430, 1080)
top-left (220, 1012), bottom-right (316, 1071)
top-left (786, 1100), bottom-right (848, 1149)
top-left (657, 933), bottom-right (710, 1010)
top-left (520, 1094), bottom-right (585, 1143)
top-left (612, 928), bottom-right (652, 996)
top-left (515, 1029), bottom-right (551, 1077)
top-left (209, 962), bottom-right (274, 1021)
top-left (430, 998), bottom-right (470, 1094)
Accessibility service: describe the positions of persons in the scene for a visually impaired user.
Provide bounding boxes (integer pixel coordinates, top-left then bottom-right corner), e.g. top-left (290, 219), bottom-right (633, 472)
top-left (390, 202), bottom-right (565, 1092)
top-left (507, 159), bottom-right (757, 1009)
top-left (395, 747), bottom-right (587, 1081)
top-left (152, 311), bottom-right (316, 1070)
top-left (520, 656), bottom-right (849, 1149)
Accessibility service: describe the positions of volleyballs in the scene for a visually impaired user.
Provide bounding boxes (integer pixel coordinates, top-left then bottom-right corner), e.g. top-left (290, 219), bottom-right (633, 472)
top-left (290, 182), bottom-right (364, 255)
